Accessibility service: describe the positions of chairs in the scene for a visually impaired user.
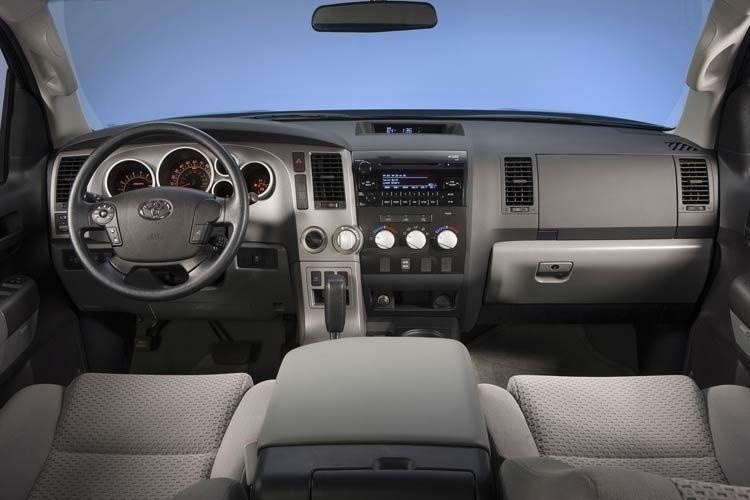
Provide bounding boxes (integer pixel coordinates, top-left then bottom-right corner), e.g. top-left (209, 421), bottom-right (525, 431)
top-left (478, 374), bottom-right (750, 500)
top-left (0, 373), bottom-right (275, 500)
